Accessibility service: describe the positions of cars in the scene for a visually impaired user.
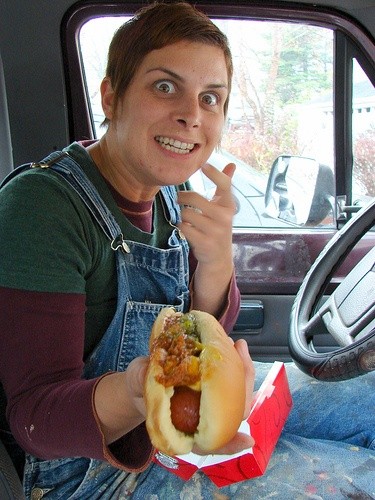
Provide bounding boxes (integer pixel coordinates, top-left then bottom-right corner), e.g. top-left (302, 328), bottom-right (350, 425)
top-left (92, 112), bottom-right (298, 228)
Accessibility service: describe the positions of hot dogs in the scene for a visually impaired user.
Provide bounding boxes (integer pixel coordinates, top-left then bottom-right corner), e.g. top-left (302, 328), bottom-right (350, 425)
top-left (144, 308), bottom-right (245, 455)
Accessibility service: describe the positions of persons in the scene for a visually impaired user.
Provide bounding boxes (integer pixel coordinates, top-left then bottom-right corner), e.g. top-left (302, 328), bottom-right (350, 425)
top-left (2, 0), bottom-right (373, 499)
